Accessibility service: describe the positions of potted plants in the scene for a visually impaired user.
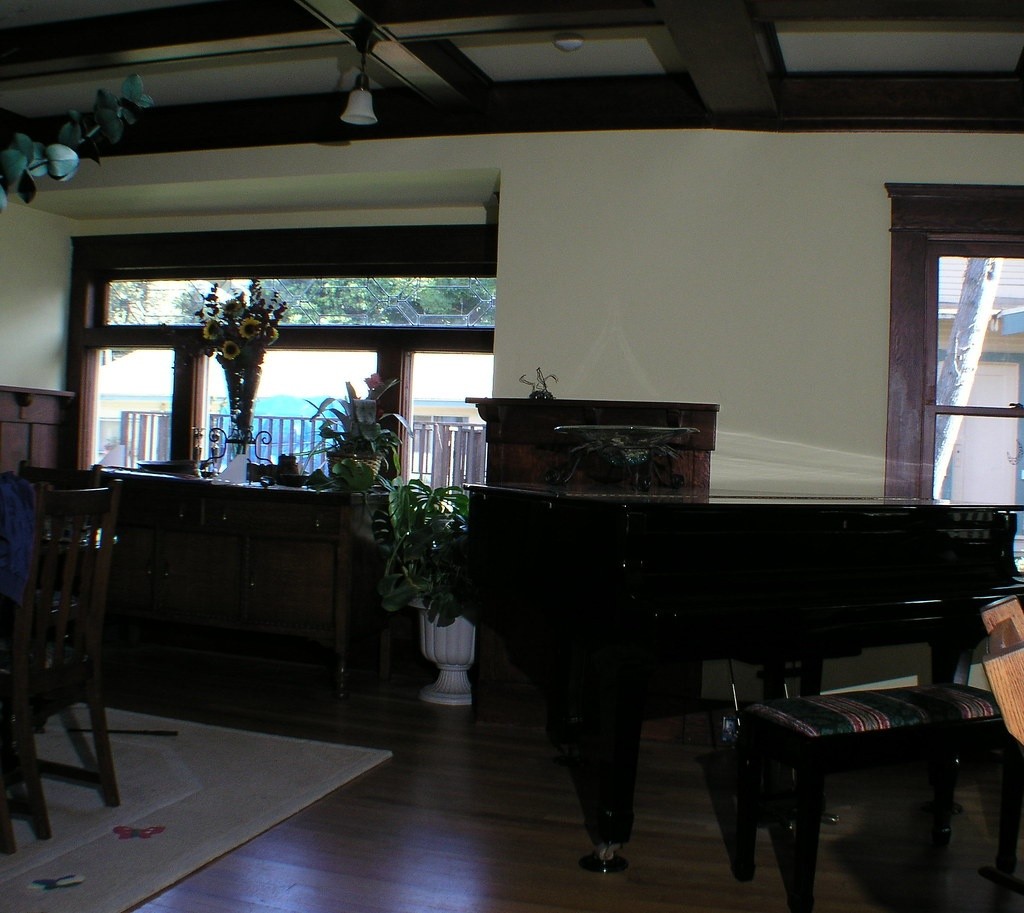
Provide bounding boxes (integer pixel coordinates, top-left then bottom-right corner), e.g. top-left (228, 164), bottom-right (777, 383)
top-left (370, 473), bottom-right (479, 707)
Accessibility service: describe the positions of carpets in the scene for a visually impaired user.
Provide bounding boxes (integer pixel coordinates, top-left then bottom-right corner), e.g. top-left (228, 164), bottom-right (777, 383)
top-left (0, 703), bottom-right (393, 913)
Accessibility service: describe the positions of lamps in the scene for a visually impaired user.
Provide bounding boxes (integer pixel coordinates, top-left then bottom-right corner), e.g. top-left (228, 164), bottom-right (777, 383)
top-left (340, 57), bottom-right (378, 126)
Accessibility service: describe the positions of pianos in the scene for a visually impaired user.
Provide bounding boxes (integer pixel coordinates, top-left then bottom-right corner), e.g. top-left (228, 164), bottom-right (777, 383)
top-left (469, 489), bottom-right (1024, 868)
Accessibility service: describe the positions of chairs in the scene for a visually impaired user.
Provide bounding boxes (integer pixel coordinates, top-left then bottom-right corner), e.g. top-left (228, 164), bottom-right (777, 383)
top-left (0, 478), bottom-right (121, 840)
top-left (15, 459), bottom-right (101, 629)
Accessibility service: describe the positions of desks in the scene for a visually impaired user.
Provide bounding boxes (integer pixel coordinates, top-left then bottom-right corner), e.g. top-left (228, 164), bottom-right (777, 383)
top-left (0, 522), bottom-right (119, 558)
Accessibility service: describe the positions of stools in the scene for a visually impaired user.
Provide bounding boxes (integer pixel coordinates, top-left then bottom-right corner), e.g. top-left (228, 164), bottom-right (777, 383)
top-left (730, 682), bottom-right (1024, 913)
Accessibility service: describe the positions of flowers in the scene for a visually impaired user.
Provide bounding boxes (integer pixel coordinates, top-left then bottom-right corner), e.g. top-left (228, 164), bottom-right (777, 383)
top-left (194, 276), bottom-right (288, 360)
top-left (293, 371), bottom-right (414, 492)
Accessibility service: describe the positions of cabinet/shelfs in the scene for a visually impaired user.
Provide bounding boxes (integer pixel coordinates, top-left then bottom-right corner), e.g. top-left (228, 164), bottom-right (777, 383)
top-left (0, 385), bottom-right (76, 477)
top-left (464, 397), bottom-right (722, 730)
top-left (99, 467), bottom-right (391, 701)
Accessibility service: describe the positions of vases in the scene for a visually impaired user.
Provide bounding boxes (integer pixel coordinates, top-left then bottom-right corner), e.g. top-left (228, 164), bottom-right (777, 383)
top-left (217, 350), bottom-right (265, 456)
top-left (325, 451), bottom-right (383, 487)
